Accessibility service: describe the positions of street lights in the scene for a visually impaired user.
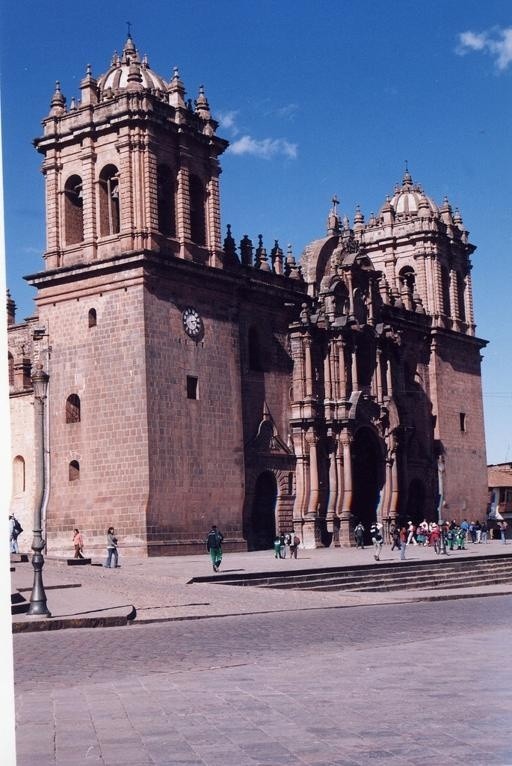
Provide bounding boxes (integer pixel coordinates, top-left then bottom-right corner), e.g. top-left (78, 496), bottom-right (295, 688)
top-left (23, 359), bottom-right (57, 617)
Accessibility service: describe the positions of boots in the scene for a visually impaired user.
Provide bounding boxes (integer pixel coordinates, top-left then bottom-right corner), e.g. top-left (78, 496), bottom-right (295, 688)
top-left (456, 546), bottom-right (465, 550)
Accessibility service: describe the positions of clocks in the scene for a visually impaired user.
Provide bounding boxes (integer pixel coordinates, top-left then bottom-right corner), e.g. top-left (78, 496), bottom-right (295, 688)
top-left (181, 305), bottom-right (202, 337)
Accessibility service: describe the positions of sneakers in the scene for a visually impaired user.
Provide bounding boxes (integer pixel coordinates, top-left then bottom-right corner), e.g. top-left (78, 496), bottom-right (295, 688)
top-left (105, 565), bottom-right (121, 568)
top-left (213, 564), bottom-right (219, 572)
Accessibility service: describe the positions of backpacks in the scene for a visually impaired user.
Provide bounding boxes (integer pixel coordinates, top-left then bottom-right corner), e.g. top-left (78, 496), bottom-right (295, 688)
top-left (208, 532), bottom-right (218, 548)
top-left (357, 526), bottom-right (363, 536)
top-left (294, 537), bottom-right (300, 545)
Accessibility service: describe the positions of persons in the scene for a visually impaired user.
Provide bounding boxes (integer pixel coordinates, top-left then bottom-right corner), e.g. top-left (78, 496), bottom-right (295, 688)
top-left (274, 529), bottom-right (297, 559)
top-left (207, 525), bottom-right (223, 571)
top-left (355, 519), bottom-right (508, 561)
top-left (72, 529), bottom-right (83, 558)
top-left (107, 527), bottom-right (121, 568)
top-left (9, 514), bottom-right (20, 554)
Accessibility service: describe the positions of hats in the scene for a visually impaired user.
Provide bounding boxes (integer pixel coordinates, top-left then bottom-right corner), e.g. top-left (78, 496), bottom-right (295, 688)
top-left (408, 521), bottom-right (412, 524)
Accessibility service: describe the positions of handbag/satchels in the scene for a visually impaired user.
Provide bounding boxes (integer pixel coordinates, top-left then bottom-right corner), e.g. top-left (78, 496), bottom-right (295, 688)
top-left (287, 540), bottom-right (291, 544)
top-left (112, 537), bottom-right (118, 546)
